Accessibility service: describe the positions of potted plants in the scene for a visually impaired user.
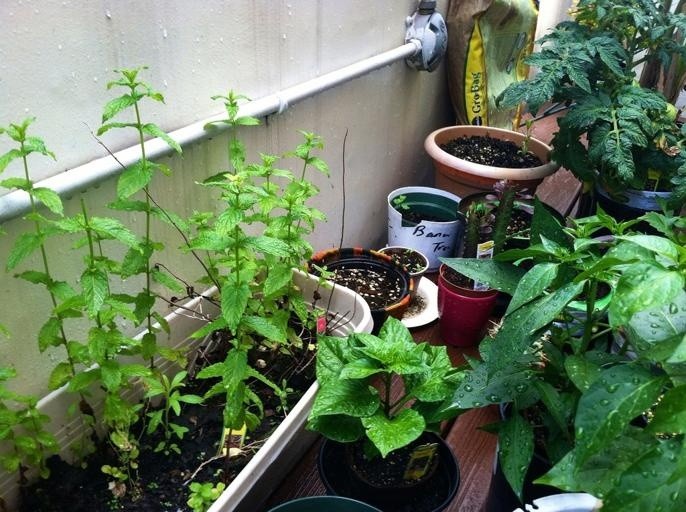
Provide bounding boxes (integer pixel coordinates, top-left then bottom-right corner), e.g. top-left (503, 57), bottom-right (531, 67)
top-left (491, 2), bottom-right (684, 234)
top-left (385, 186), bottom-right (462, 276)
top-left (304, 246), bottom-right (439, 331)
top-left (439, 260), bottom-right (499, 349)
top-left (424, 123), bottom-right (560, 257)
top-left (1, 64), bottom-right (375, 511)
top-left (305, 313), bottom-right (468, 510)
